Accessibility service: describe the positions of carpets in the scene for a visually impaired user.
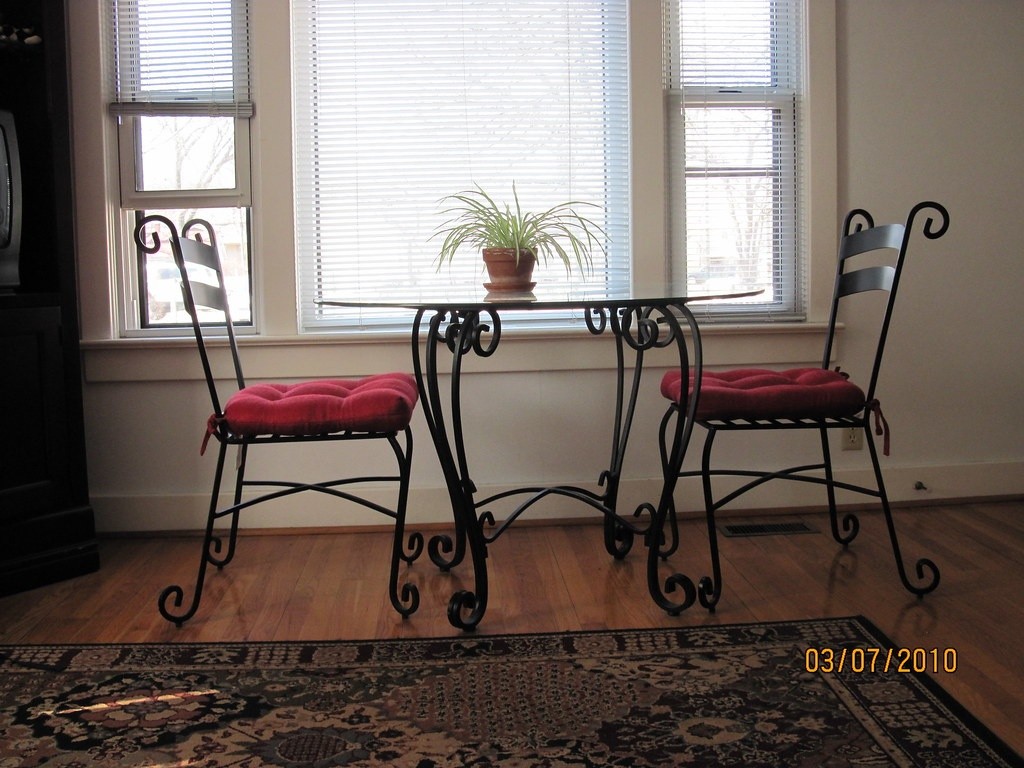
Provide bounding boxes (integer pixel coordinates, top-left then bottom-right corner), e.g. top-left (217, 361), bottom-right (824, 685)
top-left (0, 617), bottom-right (1024, 768)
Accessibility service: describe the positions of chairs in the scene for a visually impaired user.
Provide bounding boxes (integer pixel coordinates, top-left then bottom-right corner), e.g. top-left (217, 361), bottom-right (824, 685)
top-left (132, 215), bottom-right (426, 629)
top-left (659, 200), bottom-right (950, 617)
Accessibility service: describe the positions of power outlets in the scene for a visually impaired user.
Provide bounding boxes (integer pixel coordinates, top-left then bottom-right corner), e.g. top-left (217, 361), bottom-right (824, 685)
top-left (841, 427), bottom-right (864, 451)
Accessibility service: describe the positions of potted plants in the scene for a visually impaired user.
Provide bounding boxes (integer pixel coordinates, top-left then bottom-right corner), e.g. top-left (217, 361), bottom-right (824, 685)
top-left (428, 179), bottom-right (615, 294)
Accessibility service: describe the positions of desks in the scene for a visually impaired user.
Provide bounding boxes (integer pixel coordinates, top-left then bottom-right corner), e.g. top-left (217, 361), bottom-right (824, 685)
top-left (315, 287), bottom-right (766, 634)
